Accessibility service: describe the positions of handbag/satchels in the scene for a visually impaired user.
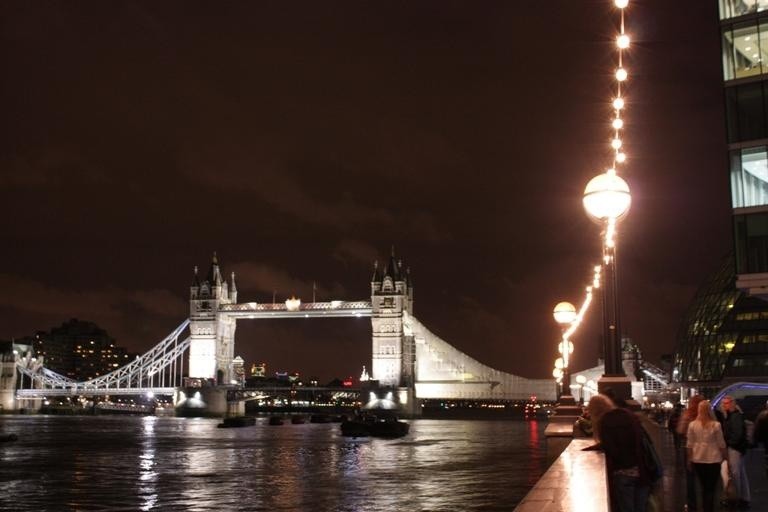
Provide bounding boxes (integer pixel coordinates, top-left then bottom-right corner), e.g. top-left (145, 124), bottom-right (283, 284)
top-left (641, 426), bottom-right (663, 480)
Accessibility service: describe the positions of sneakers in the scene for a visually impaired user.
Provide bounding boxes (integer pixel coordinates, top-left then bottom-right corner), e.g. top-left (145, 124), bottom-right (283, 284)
top-left (720, 498), bottom-right (752, 508)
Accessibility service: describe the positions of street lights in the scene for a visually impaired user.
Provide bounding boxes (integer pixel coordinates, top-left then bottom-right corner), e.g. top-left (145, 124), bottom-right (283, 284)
top-left (552, 263), bottom-right (601, 411)
top-left (581, 172), bottom-right (632, 381)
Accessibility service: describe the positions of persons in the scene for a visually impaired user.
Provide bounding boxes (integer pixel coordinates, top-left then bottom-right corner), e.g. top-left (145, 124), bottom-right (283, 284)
top-left (577, 386), bottom-right (768, 512)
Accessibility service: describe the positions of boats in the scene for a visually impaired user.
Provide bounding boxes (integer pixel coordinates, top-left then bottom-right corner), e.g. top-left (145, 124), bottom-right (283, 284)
top-left (215, 410), bottom-right (409, 440)
